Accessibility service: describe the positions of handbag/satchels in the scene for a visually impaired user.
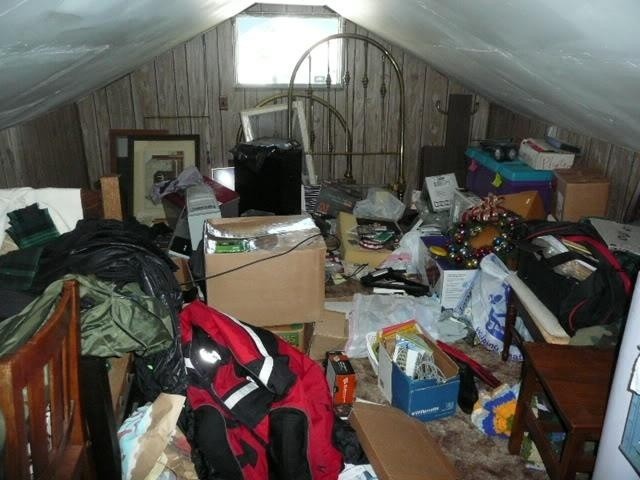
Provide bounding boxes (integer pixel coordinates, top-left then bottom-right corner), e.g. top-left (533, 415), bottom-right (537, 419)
top-left (511, 216), bottom-right (635, 337)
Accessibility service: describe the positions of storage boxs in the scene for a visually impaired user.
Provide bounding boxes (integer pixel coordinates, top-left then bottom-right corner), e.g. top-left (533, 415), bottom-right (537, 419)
top-left (376, 321), bottom-right (461, 420)
top-left (416, 139), bottom-right (611, 312)
top-left (201, 214), bottom-right (356, 418)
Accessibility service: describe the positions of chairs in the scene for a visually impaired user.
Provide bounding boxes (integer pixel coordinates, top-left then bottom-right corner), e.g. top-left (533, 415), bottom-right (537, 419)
top-left (501, 181), bottom-right (640, 480)
top-left (0, 279), bottom-right (91, 479)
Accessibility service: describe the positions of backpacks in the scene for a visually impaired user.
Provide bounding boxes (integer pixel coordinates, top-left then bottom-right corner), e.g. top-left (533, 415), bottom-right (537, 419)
top-left (177, 296), bottom-right (348, 480)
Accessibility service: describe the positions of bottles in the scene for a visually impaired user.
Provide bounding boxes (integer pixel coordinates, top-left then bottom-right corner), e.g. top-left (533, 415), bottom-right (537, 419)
top-left (428, 245), bottom-right (447, 269)
top-left (215, 243), bottom-right (248, 254)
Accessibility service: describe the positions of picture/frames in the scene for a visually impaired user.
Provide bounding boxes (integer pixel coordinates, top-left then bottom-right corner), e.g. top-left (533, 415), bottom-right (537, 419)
top-left (239, 101), bottom-right (315, 184)
top-left (108, 128), bottom-right (200, 226)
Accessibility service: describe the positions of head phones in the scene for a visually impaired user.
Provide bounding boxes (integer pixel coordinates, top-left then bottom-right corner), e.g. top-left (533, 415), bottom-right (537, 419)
top-left (492, 142), bottom-right (517, 162)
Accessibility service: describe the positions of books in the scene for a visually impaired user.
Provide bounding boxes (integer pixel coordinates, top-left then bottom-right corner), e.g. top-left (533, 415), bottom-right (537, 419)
top-left (313, 178), bottom-right (362, 218)
top-left (425, 172), bottom-right (461, 212)
